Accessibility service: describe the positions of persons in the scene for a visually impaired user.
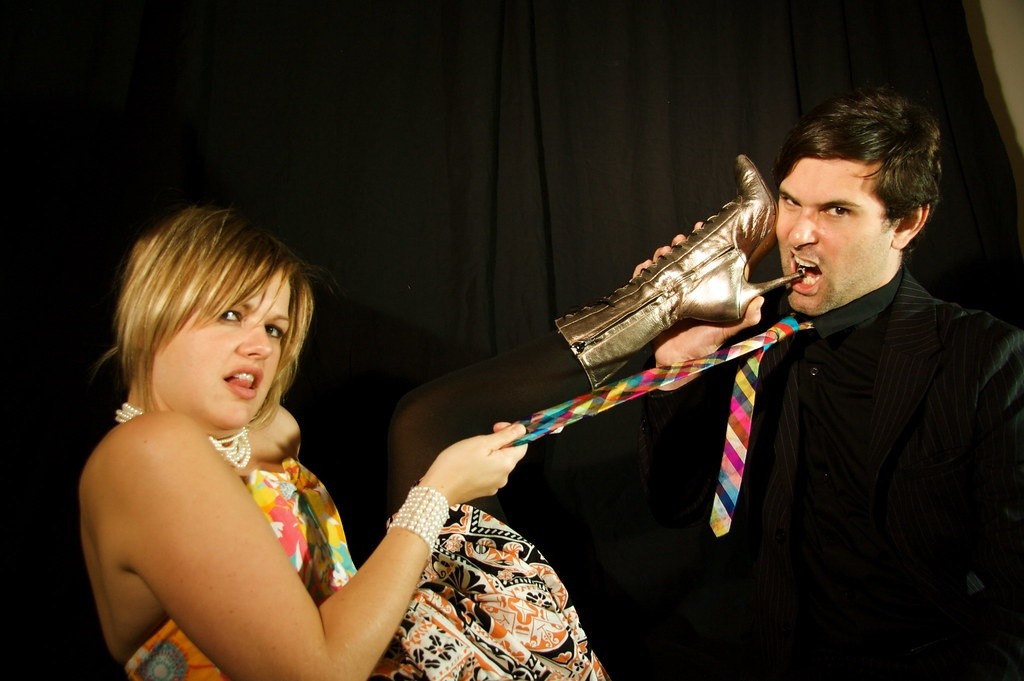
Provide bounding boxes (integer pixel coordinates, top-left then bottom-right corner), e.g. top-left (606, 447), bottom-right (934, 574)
top-left (79, 155), bottom-right (805, 681)
top-left (638, 88), bottom-right (1024, 681)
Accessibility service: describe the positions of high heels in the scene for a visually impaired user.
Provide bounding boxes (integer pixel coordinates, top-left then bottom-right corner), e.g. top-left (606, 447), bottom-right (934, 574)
top-left (553, 155), bottom-right (809, 391)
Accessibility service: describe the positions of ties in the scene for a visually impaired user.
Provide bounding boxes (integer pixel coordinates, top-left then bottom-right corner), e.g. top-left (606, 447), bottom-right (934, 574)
top-left (500, 312), bottom-right (814, 538)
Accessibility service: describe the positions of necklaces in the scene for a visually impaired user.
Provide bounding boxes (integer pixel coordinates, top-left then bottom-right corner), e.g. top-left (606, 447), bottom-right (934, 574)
top-left (113, 403), bottom-right (252, 469)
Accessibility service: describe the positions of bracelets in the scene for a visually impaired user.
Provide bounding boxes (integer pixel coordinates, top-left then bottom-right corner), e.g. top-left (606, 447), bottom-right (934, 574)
top-left (386, 486), bottom-right (450, 560)
